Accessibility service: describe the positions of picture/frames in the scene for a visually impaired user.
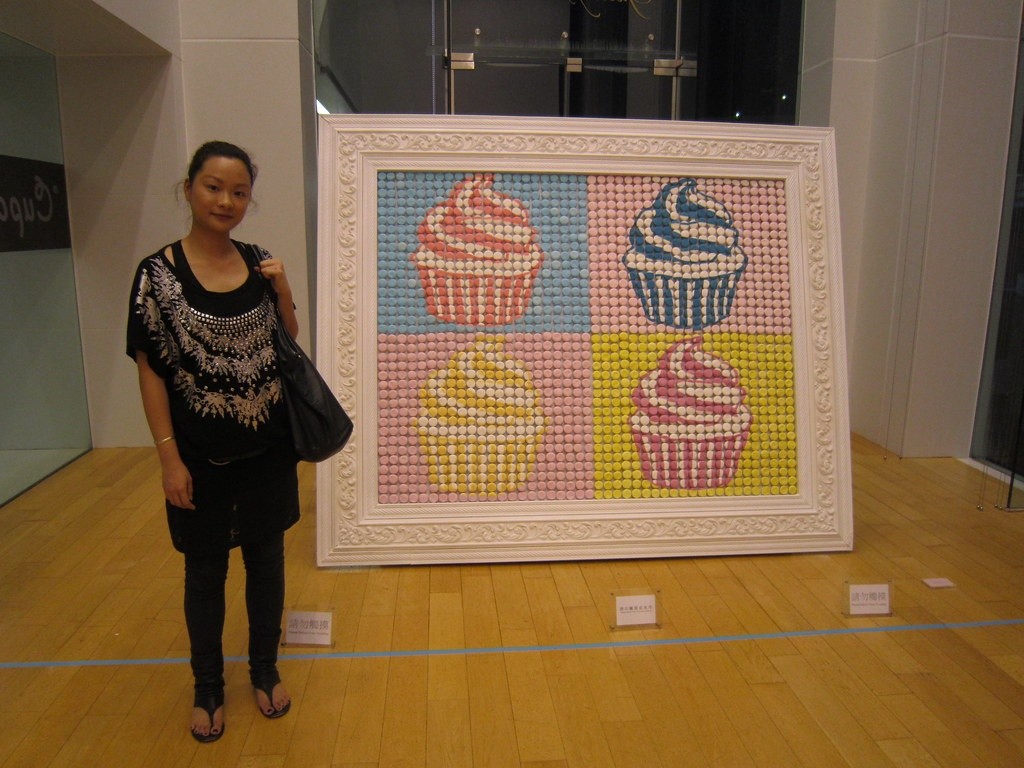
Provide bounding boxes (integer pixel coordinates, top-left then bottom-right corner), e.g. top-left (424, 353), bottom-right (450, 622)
top-left (315, 113), bottom-right (854, 568)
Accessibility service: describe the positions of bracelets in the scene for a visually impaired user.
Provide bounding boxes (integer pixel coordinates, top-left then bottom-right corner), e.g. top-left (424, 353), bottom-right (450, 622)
top-left (153, 435), bottom-right (175, 445)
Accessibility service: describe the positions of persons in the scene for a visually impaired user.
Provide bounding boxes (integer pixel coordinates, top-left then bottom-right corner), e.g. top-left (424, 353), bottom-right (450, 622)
top-left (126, 140), bottom-right (299, 742)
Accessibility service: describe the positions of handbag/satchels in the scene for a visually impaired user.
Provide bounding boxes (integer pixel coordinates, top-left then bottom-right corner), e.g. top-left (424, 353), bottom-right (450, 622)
top-left (249, 242), bottom-right (353, 464)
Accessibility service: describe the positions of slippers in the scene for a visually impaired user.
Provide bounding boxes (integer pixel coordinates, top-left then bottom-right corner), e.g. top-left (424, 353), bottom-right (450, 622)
top-left (190, 681), bottom-right (224, 742)
top-left (250, 673), bottom-right (290, 718)
